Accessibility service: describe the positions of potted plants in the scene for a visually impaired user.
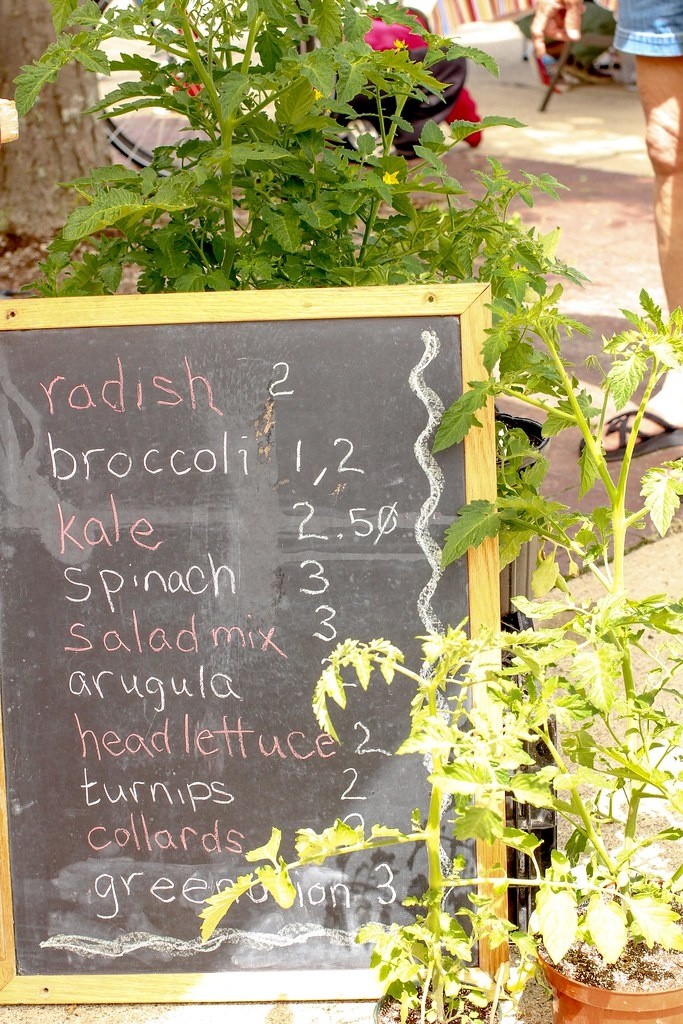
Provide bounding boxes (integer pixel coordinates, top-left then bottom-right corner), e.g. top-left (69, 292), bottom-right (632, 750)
top-left (191, 256), bottom-right (683, 1024)
top-left (12, 0), bottom-right (553, 644)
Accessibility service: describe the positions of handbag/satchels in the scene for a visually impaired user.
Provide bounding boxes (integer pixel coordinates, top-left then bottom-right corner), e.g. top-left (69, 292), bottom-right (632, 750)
top-left (325, 42), bottom-right (468, 166)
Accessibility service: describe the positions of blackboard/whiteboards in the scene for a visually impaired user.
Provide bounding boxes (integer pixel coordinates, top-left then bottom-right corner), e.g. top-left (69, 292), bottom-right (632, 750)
top-left (1, 282), bottom-right (509, 1012)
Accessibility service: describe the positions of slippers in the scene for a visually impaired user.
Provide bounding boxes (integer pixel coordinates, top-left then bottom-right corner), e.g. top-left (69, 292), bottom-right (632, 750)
top-left (578, 410), bottom-right (683, 461)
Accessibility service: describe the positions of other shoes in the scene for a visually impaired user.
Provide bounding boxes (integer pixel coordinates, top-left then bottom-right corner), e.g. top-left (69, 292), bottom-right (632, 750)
top-left (494, 402), bottom-right (552, 471)
top-left (561, 53), bottom-right (614, 84)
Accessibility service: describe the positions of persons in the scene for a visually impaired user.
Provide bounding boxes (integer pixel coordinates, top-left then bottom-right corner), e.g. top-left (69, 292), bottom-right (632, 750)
top-left (529, 0), bottom-right (683, 462)
